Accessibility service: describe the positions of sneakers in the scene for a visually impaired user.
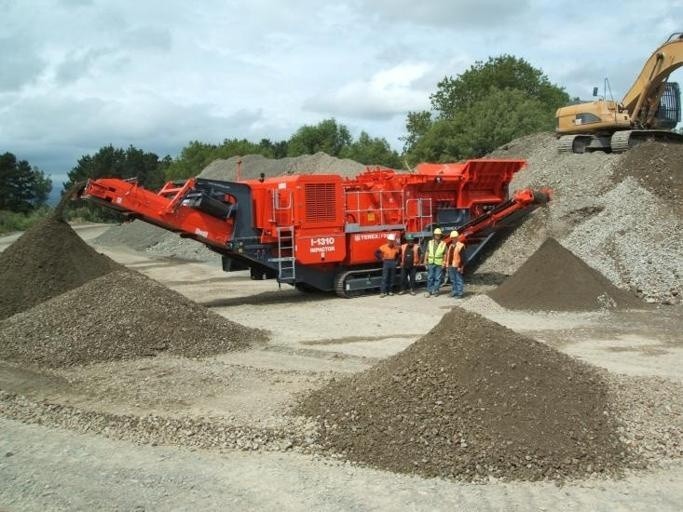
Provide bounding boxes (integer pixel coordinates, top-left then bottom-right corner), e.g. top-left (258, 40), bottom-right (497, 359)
top-left (451, 294), bottom-right (463, 299)
top-left (398, 289), bottom-right (416, 296)
top-left (425, 291), bottom-right (440, 298)
top-left (379, 291), bottom-right (394, 298)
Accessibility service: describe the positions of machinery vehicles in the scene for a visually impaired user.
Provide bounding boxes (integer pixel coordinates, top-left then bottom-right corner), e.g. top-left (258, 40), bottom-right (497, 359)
top-left (70, 158), bottom-right (553, 298)
top-left (555, 32), bottom-right (683, 156)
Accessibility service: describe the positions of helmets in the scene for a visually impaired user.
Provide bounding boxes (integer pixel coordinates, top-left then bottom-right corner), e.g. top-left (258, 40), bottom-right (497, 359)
top-left (434, 228), bottom-right (443, 235)
top-left (406, 233), bottom-right (414, 240)
top-left (387, 234), bottom-right (396, 241)
top-left (449, 231), bottom-right (459, 238)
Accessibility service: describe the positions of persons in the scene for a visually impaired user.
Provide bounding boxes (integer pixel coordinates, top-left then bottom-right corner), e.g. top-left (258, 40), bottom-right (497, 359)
top-left (445, 230), bottom-right (466, 298)
top-left (423, 227), bottom-right (448, 299)
top-left (373, 233), bottom-right (402, 298)
top-left (398, 234), bottom-right (423, 295)
